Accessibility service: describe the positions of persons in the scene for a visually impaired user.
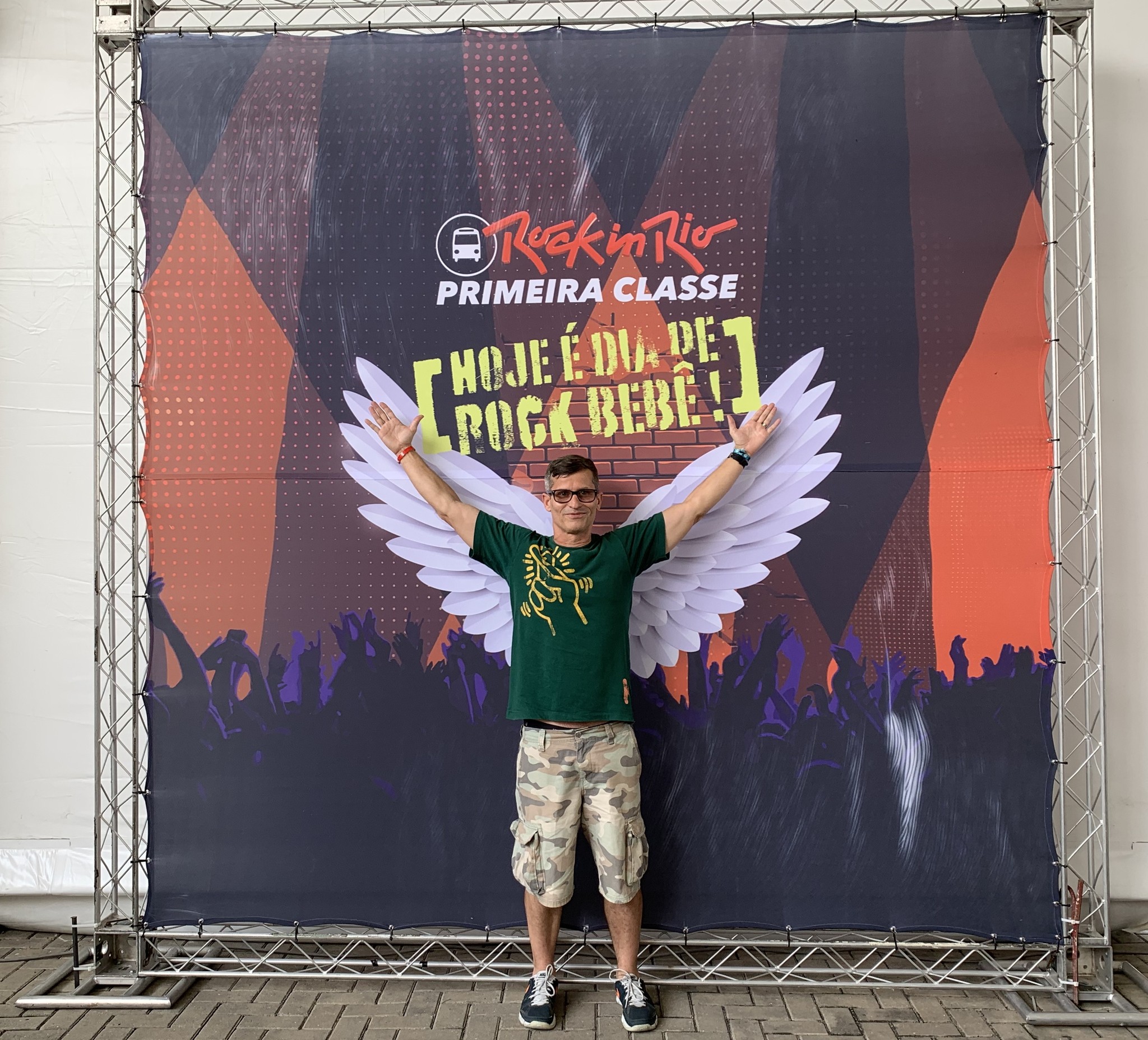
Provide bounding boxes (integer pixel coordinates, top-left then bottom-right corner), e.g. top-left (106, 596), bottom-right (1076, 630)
top-left (366, 400), bottom-right (782, 1031)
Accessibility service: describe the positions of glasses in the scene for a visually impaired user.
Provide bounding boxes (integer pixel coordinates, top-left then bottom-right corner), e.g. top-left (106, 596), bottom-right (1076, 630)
top-left (546, 489), bottom-right (599, 503)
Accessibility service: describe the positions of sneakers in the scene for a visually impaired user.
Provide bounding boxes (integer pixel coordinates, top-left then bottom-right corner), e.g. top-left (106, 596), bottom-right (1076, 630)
top-left (518, 963), bottom-right (559, 1030)
top-left (609, 969), bottom-right (658, 1032)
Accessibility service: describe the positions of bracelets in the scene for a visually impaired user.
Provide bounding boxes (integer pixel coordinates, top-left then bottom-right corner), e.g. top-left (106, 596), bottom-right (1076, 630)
top-left (397, 446), bottom-right (415, 465)
top-left (727, 448), bottom-right (750, 469)
